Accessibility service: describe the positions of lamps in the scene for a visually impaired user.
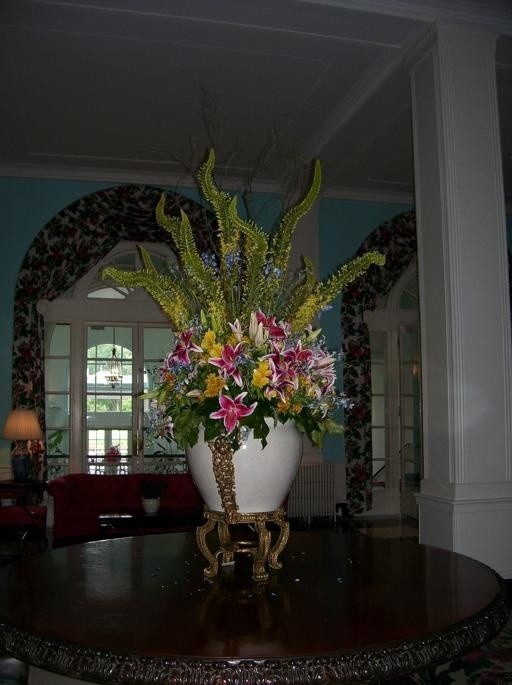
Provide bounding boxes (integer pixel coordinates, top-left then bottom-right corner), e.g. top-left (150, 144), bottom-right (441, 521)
top-left (1, 409), bottom-right (43, 484)
top-left (104, 326), bottom-right (122, 390)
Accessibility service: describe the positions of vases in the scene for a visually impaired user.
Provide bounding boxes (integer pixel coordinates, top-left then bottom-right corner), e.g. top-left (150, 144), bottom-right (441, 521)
top-left (185, 416), bottom-right (304, 513)
top-left (140, 496), bottom-right (160, 514)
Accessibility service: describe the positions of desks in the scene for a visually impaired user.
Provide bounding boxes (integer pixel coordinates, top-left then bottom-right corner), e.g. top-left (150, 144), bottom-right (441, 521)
top-left (0, 530), bottom-right (512, 685)
top-left (0, 479), bottom-right (47, 556)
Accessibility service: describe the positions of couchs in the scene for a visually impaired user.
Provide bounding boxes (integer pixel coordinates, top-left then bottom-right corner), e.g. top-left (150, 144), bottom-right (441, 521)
top-left (47, 473), bottom-right (205, 549)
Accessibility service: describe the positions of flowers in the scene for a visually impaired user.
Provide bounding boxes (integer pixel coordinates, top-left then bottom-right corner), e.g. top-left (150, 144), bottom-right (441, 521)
top-left (101, 147), bottom-right (387, 451)
